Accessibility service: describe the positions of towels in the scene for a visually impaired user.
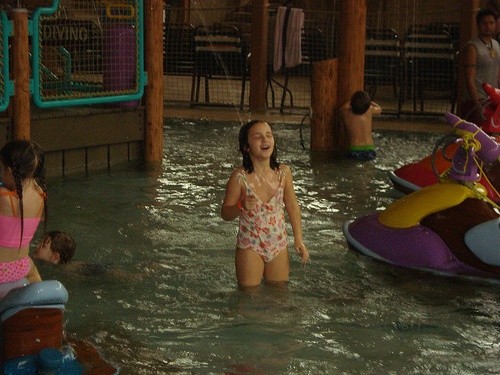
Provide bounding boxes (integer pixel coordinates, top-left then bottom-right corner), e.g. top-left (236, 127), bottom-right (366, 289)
top-left (273, 7), bottom-right (286, 73)
top-left (282, 8), bottom-right (304, 68)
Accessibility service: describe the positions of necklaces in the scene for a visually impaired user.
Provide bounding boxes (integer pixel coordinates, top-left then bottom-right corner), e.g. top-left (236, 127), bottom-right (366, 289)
top-left (479, 36), bottom-right (496, 58)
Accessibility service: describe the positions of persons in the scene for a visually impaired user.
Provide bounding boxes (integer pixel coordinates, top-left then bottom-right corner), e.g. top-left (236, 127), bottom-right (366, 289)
top-left (221, 292), bottom-right (307, 375)
top-left (337, 90), bottom-right (383, 159)
top-left (32, 231), bottom-right (158, 285)
top-left (0, 139), bottom-right (48, 284)
top-left (459, 9), bottom-right (500, 119)
top-left (221, 120), bottom-right (308, 291)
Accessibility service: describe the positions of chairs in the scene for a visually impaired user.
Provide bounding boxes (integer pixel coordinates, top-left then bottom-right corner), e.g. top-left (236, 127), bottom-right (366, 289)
top-left (162, 23), bottom-right (196, 103)
top-left (365, 21), bottom-right (459, 122)
top-left (279, 27), bottom-right (323, 116)
top-left (189, 24), bottom-right (251, 111)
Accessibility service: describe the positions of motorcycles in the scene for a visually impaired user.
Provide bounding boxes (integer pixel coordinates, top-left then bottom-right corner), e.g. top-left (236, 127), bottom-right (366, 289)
top-left (388, 83), bottom-right (500, 205)
top-left (343, 113), bottom-right (500, 284)
